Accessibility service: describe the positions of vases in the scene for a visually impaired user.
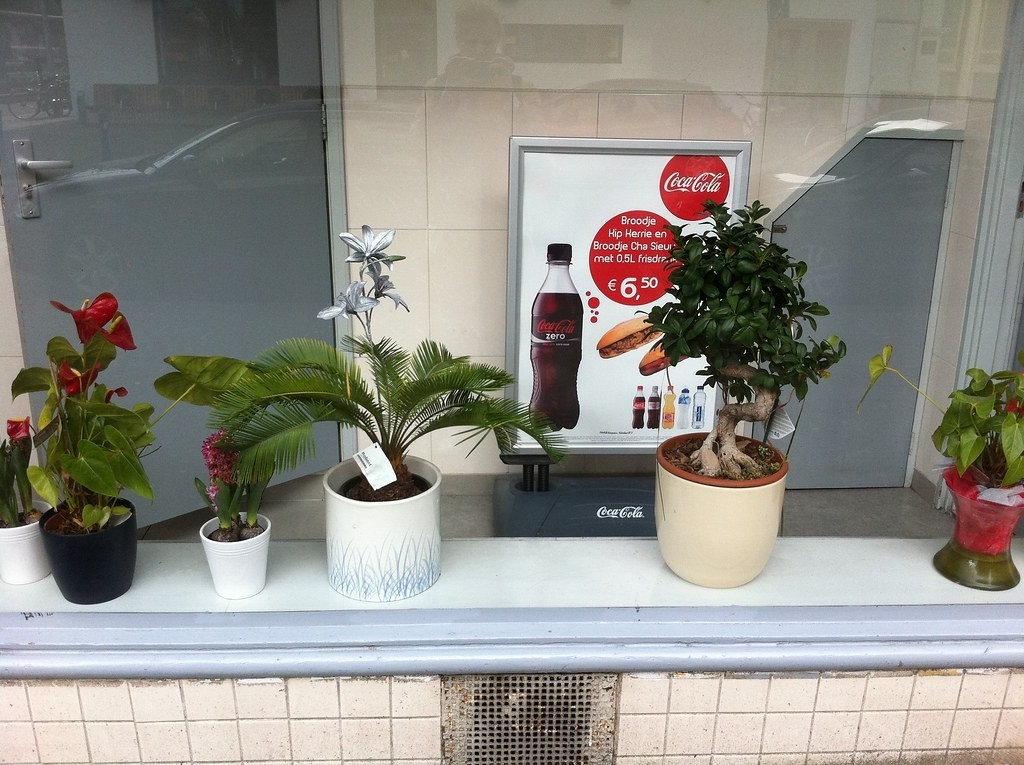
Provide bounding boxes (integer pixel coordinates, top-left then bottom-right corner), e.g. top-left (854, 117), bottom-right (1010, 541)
top-left (40, 495), bottom-right (138, 605)
top-left (199, 512), bottom-right (270, 598)
top-left (320, 453), bottom-right (441, 602)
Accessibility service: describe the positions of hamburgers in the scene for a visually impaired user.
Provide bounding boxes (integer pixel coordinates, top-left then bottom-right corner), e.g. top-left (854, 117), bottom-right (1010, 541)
top-left (596, 315), bottom-right (663, 359)
top-left (639, 345), bottom-right (689, 376)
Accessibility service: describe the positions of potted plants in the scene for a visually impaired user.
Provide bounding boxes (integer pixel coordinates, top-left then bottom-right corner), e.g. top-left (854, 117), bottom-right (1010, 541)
top-left (630, 202), bottom-right (849, 590)
top-left (0, 436), bottom-right (51, 584)
top-left (858, 344), bottom-right (1024, 593)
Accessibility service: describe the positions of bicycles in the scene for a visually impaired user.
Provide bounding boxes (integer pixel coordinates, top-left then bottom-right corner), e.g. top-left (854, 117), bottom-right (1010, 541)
top-left (6, 55), bottom-right (73, 122)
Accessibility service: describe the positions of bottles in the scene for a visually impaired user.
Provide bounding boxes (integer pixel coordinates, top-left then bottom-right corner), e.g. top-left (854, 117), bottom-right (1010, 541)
top-left (647, 386), bottom-right (660, 429)
top-left (691, 386), bottom-right (706, 429)
top-left (662, 386), bottom-right (675, 429)
top-left (632, 386), bottom-right (645, 428)
top-left (676, 388), bottom-right (691, 429)
top-left (529, 243), bottom-right (584, 431)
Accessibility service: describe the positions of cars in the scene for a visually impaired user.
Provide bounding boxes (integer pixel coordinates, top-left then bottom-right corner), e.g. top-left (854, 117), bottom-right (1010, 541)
top-left (757, 120), bottom-right (966, 489)
top-left (2, 99), bottom-right (335, 371)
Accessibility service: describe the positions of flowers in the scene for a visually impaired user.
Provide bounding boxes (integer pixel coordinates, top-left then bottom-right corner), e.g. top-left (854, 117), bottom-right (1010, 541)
top-left (6, 290), bottom-right (156, 528)
top-left (203, 224), bottom-right (566, 486)
top-left (194, 429), bottom-right (275, 531)
